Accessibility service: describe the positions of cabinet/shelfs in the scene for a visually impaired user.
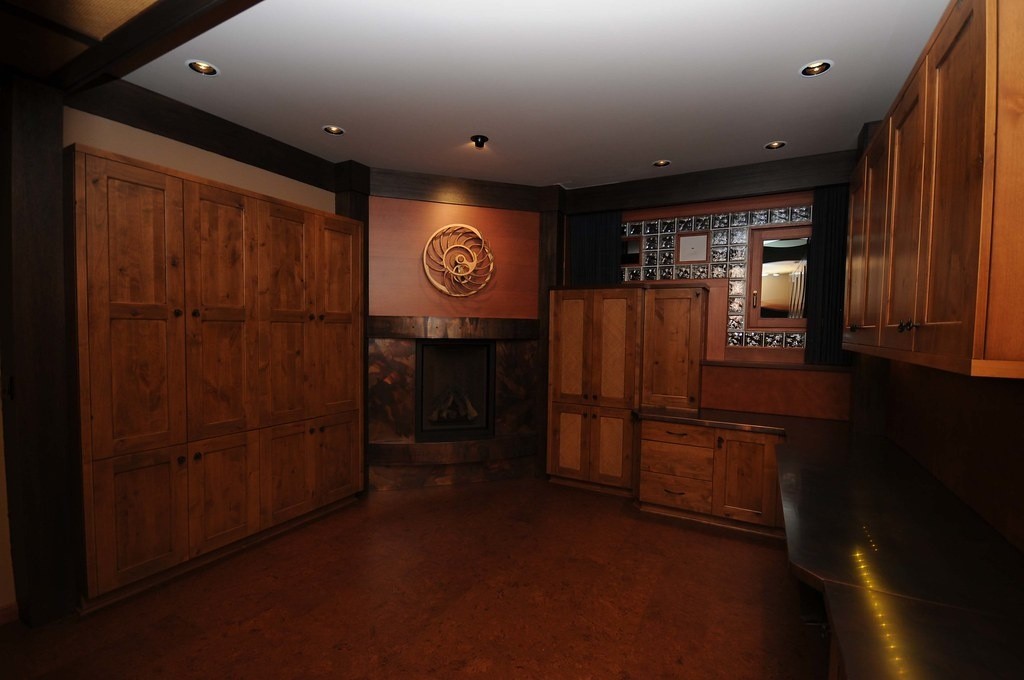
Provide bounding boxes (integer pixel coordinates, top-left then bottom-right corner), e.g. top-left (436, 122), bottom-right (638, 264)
top-left (544, 285), bottom-right (789, 545)
top-left (842, 0), bottom-right (1024, 379)
top-left (59, 144), bottom-right (367, 617)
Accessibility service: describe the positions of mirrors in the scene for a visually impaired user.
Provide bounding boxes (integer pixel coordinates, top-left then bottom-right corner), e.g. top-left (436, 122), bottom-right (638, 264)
top-left (748, 226), bottom-right (808, 328)
